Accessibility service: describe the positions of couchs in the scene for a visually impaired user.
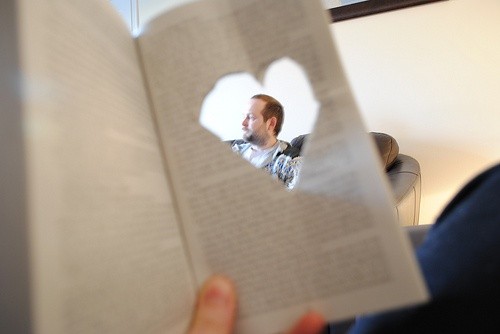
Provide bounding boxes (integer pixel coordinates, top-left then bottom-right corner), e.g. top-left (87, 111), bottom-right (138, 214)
top-left (291, 132), bottom-right (422, 228)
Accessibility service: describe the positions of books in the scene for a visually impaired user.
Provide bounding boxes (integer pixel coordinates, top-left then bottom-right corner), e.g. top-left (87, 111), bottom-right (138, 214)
top-left (1, 0), bottom-right (433, 334)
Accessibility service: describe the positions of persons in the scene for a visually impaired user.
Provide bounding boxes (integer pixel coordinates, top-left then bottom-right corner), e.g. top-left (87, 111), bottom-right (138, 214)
top-left (190, 275), bottom-right (323, 334)
top-left (228, 94), bottom-right (300, 187)
